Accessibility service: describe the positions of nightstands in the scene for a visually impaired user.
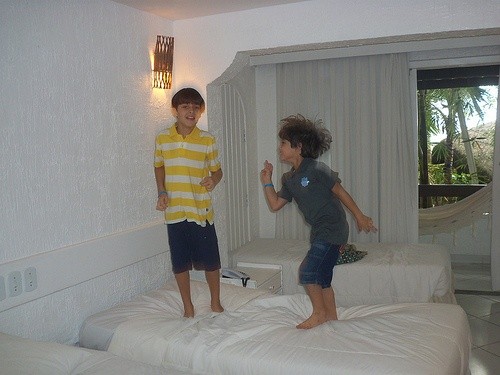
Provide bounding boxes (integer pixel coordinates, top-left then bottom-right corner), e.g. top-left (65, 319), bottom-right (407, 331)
top-left (219, 263), bottom-right (283, 297)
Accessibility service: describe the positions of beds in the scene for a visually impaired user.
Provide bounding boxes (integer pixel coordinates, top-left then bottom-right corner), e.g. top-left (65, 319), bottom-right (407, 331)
top-left (0, 334), bottom-right (187, 375)
top-left (235, 238), bottom-right (457, 310)
top-left (79, 279), bottom-right (471, 375)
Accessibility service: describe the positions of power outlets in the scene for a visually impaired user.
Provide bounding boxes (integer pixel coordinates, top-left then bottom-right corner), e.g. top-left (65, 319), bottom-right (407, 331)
top-left (9, 272), bottom-right (23, 296)
top-left (24, 266), bottom-right (38, 291)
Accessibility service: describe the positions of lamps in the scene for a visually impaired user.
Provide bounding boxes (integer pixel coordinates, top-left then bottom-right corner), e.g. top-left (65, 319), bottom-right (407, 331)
top-left (153, 35), bottom-right (174, 90)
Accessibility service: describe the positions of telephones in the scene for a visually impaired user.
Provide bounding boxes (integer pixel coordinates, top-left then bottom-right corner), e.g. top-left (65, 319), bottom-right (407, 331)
top-left (222, 269), bottom-right (250, 280)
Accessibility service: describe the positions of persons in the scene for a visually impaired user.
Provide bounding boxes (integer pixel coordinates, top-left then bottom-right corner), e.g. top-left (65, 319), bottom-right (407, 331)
top-left (260, 114), bottom-right (377, 329)
top-left (154, 88), bottom-right (223, 319)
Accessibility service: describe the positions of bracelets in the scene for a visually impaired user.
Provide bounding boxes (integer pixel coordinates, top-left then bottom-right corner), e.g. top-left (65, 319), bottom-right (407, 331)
top-left (262, 179), bottom-right (275, 190)
top-left (157, 191), bottom-right (168, 198)
